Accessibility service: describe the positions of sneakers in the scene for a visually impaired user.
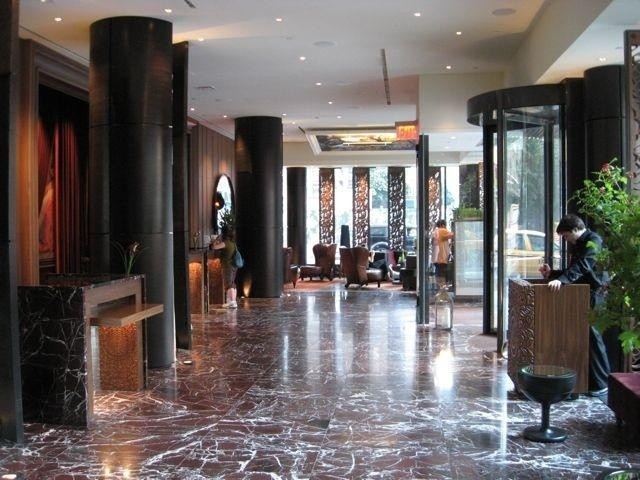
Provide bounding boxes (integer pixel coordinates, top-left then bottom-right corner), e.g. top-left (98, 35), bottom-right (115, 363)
top-left (222, 301), bottom-right (238, 309)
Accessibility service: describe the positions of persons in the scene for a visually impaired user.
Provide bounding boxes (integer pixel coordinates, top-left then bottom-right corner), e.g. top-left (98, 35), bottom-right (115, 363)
top-left (210, 224), bottom-right (239, 309)
top-left (537, 214), bottom-right (613, 397)
top-left (432, 220), bottom-right (441, 279)
top-left (436, 219), bottom-right (455, 285)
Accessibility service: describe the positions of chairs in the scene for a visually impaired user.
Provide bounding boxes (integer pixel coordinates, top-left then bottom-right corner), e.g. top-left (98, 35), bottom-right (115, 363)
top-left (282, 243), bottom-right (416, 288)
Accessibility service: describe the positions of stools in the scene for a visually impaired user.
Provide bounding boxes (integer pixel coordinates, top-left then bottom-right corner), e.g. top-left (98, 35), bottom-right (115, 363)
top-left (518, 363), bottom-right (577, 442)
top-left (607, 373), bottom-right (640, 427)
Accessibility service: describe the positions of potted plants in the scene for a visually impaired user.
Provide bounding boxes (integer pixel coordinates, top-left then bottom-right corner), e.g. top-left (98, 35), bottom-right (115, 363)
top-left (453, 207), bottom-right (483, 221)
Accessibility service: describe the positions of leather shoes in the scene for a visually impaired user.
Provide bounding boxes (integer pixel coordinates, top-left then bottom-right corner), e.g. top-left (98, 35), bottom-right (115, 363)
top-left (589, 387), bottom-right (608, 397)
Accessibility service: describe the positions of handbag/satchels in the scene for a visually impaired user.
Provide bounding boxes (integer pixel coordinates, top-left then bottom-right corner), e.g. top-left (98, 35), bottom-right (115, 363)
top-left (233, 250), bottom-right (244, 268)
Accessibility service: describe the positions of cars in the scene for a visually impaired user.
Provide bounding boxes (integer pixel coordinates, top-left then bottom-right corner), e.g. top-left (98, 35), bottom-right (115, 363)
top-left (481, 229), bottom-right (570, 279)
top-left (369, 225), bottom-right (416, 252)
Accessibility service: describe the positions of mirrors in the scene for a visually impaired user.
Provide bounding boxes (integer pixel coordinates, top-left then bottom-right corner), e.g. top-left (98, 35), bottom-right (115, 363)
top-left (213, 174), bottom-right (236, 235)
